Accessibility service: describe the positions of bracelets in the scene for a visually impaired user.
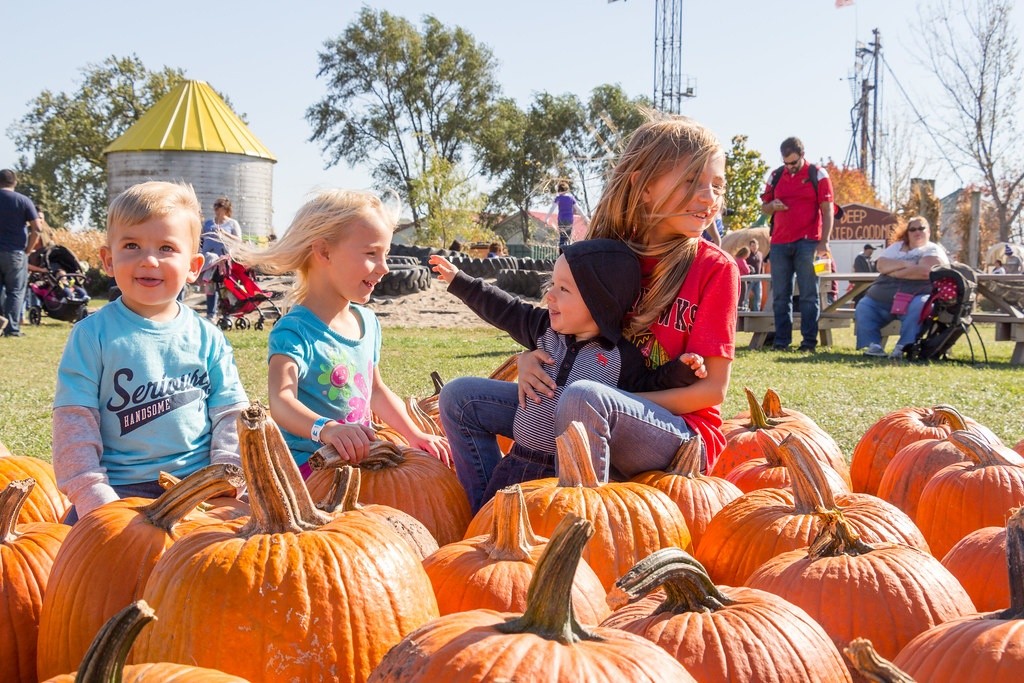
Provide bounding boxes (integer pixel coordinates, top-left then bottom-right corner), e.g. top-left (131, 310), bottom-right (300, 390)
top-left (310, 416), bottom-right (337, 446)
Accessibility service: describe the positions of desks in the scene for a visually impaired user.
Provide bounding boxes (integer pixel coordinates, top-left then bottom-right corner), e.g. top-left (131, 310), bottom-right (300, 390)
top-left (740, 273), bottom-right (1024, 364)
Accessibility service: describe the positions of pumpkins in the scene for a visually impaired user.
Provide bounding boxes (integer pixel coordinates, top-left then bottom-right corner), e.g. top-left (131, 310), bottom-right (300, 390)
top-left (0, 348), bottom-right (1024, 683)
top-left (717, 213), bottom-right (772, 311)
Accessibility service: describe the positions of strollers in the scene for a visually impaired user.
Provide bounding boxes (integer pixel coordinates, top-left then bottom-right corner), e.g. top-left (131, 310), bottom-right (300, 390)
top-left (25, 241), bottom-right (93, 324)
top-left (906, 263), bottom-right (977, 361)
top-left (201, 253), bottom-right (286, 331)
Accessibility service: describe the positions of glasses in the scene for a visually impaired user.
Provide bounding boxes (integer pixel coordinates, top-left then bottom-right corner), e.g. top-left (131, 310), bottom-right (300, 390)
top-left (783, 155), bottom-right (801, 165)
top-left (213, 202), bottom-right (224, 208)
top-left (907, 226), bottom-right (928, 232)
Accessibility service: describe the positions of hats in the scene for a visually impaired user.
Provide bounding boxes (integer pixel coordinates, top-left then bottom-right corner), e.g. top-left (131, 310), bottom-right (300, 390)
top-left (864, 244), bottom-right (877, 250)
top-left (1004, 245), bottom-right (1013, 254)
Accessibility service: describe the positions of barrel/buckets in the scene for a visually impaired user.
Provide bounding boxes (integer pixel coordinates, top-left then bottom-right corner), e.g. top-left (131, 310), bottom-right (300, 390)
top-left (812, 249), bottom-right (833, 276)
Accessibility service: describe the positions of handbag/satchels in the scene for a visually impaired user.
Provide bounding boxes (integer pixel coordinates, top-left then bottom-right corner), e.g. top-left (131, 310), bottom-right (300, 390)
top-left (890, 291), bottom-right (914, 315)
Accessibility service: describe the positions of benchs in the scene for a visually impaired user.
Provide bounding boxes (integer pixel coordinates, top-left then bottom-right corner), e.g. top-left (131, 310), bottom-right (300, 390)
top-left (736, 309), bottom-right (1024, 323)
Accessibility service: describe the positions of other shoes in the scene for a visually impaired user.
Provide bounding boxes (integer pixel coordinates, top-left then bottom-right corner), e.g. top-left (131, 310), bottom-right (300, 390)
top-left (993, 309), bottom-right (1000, 313)
top-left (862, 342), bottom-right (889, 357)
top-left (205, 313), bottom-right (216, 323)
top-left (769, 344), bottom-right (788, 352)
top-left (794, 344), bottom-right (816, 354)
top-left (4, 332), bottom-right (24, 337)
top-left (888, 345), bottom-right (904, 359)
top-left (737, 306), bottom-right (750, 311)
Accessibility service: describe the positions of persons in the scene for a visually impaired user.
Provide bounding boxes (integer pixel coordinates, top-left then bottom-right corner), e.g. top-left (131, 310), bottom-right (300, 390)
top-left (854, 212), bottom-right (952, 364)
top-left (546, 180), bottom-right (591, 247)
top-left (1004, 243), bottom-right (1024, 275)
top-left (54, 267), bottom-right (77, 299)
top-left (215, 191), bottom-right (455, 485)
top-left (854, 243), bottom-right (877, 308)
top-left (201, 195), bottom-right (241, 325)
top-left (0, 169), bottom-right (43, 338)
top-left (733, 237), bottom-right (762, 310)
top-left (760, 136), bottom-right (835, 359)
top-left (52, 182), bottom-right (251, 528)
top-left (25, 204), bottom-right (57, 274)
top-left (487, 241), bottom-right (510, 259)
top-left (429, 237), bottom-right (709, 516)
top-left (816, 236), bottom-right (839, 310)
top-left (439, 119), bottom-right (740, 514)
top-left (992, 260), bottom-right (1006, 274)
